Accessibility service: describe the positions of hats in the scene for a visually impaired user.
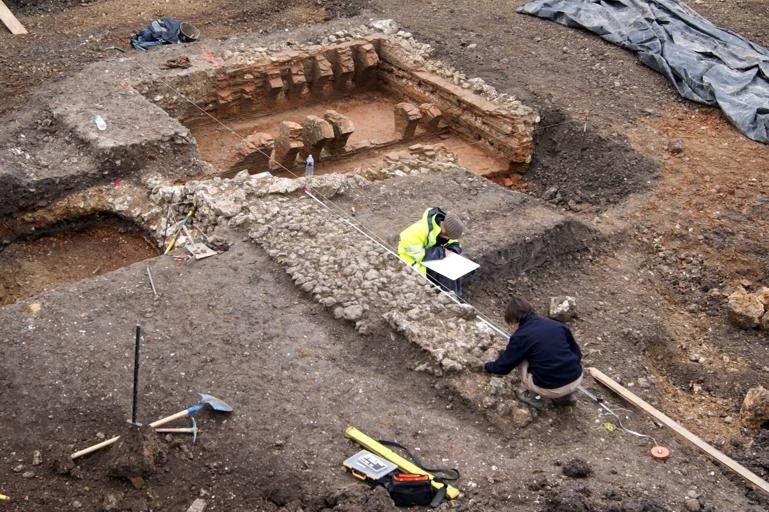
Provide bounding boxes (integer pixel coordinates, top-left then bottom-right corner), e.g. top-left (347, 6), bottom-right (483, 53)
top-left (444, 214), bottom-right (463, 239)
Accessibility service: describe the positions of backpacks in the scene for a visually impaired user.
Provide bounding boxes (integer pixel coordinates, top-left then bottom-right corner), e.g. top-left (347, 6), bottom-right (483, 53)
top-left (130, 18), bottom-right (186, 51)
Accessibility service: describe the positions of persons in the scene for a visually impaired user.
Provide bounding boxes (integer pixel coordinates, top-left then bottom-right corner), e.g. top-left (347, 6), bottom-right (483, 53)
top-left (466, 295), bottom-right (584, 407)
top-left (397, 205), bottom-right (465, 298)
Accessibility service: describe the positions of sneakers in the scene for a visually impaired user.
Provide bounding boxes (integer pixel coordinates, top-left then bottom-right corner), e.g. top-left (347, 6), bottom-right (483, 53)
top-left (514, 389), bottom-right (544, 408)
top-left (552, 392), bottom-right (577, 406)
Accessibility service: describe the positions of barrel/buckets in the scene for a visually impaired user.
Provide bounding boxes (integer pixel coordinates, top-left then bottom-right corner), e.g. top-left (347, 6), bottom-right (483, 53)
top-left (177, 21), bottom-right (202, 43)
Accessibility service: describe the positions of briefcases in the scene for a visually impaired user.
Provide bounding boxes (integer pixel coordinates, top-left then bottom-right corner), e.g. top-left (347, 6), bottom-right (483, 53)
top-left (342, 449), bottom-right (400, 481)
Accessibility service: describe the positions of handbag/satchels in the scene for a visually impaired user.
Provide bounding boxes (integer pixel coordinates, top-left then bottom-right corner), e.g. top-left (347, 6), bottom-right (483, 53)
top-left (367, 440), bottom-right (459, 507)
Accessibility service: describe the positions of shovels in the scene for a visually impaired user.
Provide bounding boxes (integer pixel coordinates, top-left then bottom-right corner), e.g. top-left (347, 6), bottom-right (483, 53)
top-left (70, 394), bottom-right (233, 460)
top-left (183, 224), bottom-right (234, 259)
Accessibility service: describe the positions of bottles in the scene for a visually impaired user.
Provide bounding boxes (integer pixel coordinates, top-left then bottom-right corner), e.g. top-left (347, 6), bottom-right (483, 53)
top-left (305, 154), bottom-right (314, 178)
